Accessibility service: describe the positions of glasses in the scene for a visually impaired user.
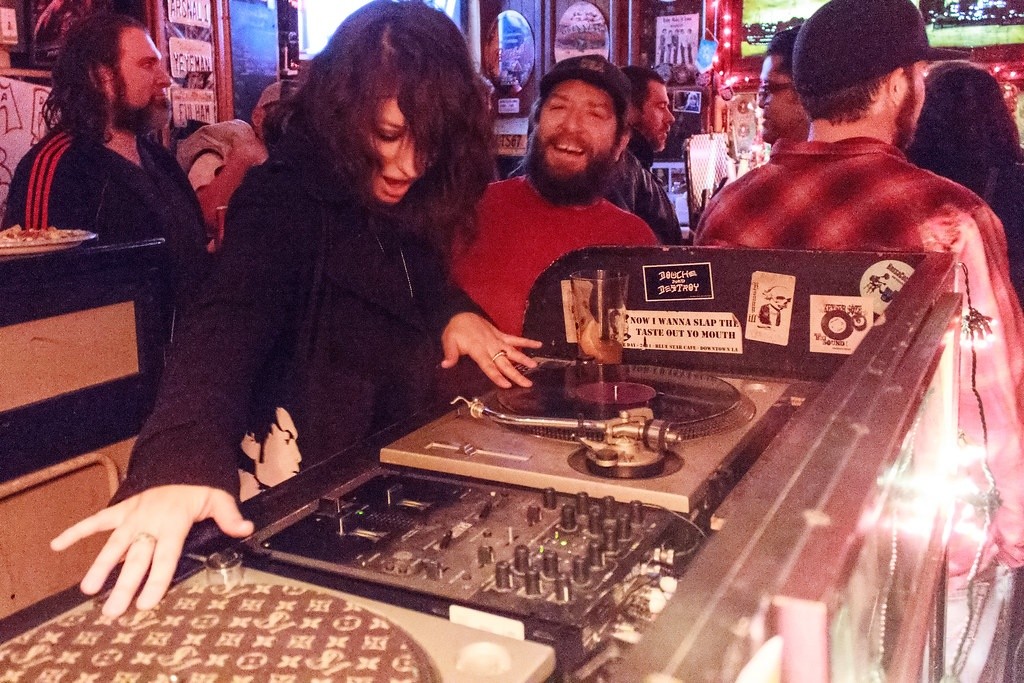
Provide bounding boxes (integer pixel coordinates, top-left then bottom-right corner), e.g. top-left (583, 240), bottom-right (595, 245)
top-left (753, 79), bottom-right (795, 99)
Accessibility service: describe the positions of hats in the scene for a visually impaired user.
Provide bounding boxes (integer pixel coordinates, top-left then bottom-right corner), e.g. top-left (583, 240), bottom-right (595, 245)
top-left (256, 77), bottom-right (302, 109)
top-left (535, 53), bottom-right (632, 110)
top-left (795, 0), bottom-right (971, 92)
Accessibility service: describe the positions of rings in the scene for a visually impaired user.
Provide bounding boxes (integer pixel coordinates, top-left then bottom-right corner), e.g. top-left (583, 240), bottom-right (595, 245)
top-left (131, 533), bottom-right (157, 545)
top-left (492, 351), bottom-right (505, 361)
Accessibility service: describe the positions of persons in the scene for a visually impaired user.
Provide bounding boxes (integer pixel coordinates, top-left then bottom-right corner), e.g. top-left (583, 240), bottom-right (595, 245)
top-left (187, 80), bottom-right (301, 255)
top-left (50, 0), bottom-right (542, 619)
top-left (758, 39), bottom-right (811, 144)
top-left (450, 56), bottom-right (659, 368)
top-left (605, 66), bottom-right (684, 247)
top-left (694, 39), bottom-right (1022, 683)
top-left (905, 60), bottom-right (1022, 309)
top-left (2, 12), bottom-right (209, 345)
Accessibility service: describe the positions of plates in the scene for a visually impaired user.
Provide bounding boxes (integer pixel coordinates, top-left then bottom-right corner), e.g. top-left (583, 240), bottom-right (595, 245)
top-left (1, 230), bottom-right (98, 256)
